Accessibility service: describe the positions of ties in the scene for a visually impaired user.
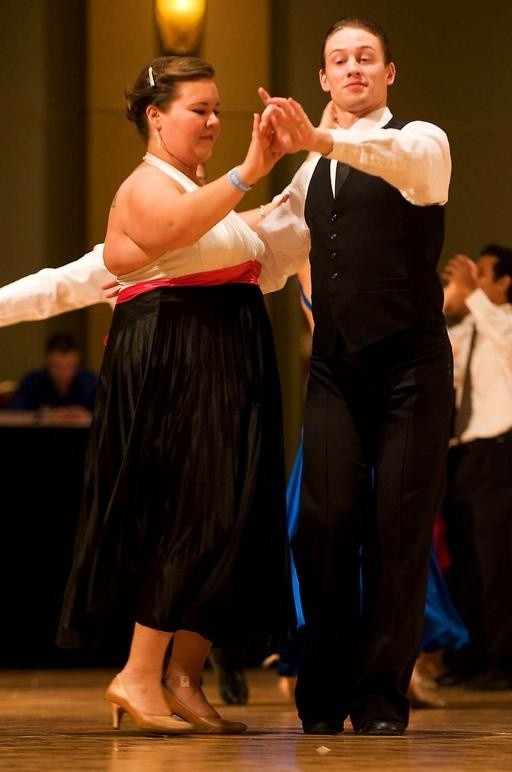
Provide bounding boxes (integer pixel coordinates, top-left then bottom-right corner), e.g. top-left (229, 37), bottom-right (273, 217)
top-left (453, 323), bottom-right (477, 437)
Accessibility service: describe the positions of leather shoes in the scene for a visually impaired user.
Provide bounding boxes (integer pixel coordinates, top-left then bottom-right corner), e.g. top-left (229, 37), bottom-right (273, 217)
top-left (408, 671), bottom-right (446, 707)
top-left (211, 649), bottom-right (249, 703)
top-left (355, 714), bottom-right (404, 737)
top-left (302, 715), bottom-right (343, 736)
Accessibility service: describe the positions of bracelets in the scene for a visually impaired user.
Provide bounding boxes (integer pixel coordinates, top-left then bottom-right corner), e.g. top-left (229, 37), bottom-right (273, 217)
top-left (258, 203), bottom-right (266, 217)
top-left (226, 164), bottom-right (255, 192)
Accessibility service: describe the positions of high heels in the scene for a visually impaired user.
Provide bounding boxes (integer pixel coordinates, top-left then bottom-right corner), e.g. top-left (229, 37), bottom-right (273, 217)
top-left (106, 672), bottom-right (194, 734)
top-left (159, 676), bottom-right (248, 735)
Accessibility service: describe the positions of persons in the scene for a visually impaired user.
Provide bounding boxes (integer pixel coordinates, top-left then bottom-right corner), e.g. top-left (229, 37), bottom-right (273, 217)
top-left (282, 260), bottom-right (468, 710)
top-left (91, 54), bottom-right (294, 734)
top-left (442, 252), bottom-right (479, 318)
top-left (441, 243), bottom-right (511, 693)
top-left (0, 162), bottom-right (290, 705)
top-left (0, 326), bottom-right (100, 428)
top-left (102, 15), bottom-right (456, 734)
